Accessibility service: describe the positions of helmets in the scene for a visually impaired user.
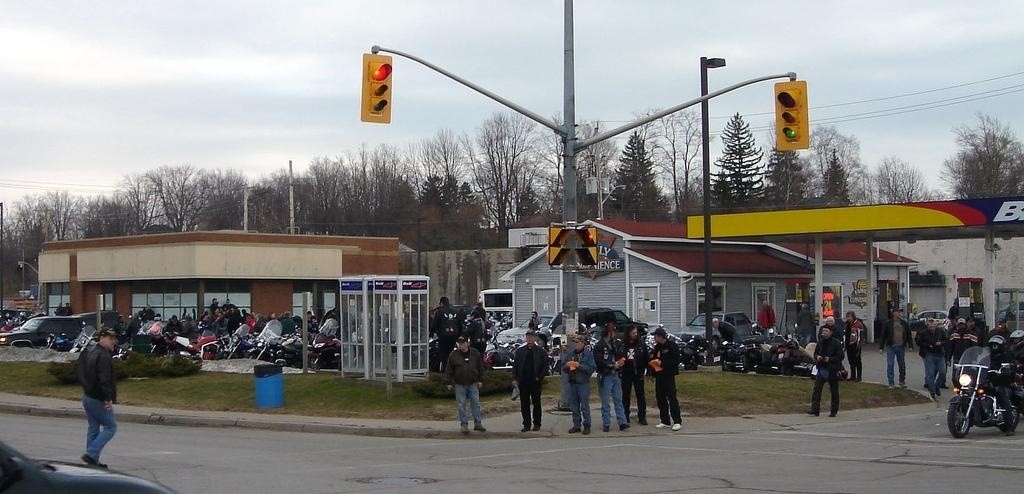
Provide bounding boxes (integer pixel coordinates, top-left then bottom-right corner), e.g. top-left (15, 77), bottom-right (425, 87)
top-left (987, 334), bottom-right (1006, 345)
top-left (957, 317), bottom-right (967, 326)
top-left (1009, 329), bottom-right (1024, 337)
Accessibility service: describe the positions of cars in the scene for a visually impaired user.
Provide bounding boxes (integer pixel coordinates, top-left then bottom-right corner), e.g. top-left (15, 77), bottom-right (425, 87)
top-left (430, 286), bottom-right (636, 350)
top-left (911, 301), bottom-right (1024, 329)
top-left (679, 309), bottom-right (753, 350)
top-left (0, 441), bottom-right (175, 492)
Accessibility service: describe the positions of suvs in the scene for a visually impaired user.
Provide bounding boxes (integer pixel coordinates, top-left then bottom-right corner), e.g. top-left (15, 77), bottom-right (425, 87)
top-left (0, 309), bottom-right (83, 353)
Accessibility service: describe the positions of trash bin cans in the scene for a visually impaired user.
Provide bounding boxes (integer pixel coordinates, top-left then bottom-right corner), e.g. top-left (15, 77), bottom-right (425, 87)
top-left (132, 333), bottom-right (151, 358)
top-left (254, 364), bottom-right (284, 408)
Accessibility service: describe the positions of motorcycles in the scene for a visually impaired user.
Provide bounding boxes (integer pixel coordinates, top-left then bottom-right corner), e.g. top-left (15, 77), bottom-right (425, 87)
top-left (943, 343), bottom-right (1021, 438)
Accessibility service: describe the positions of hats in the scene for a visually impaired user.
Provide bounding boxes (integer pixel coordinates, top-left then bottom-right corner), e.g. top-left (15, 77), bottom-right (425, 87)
top-left (525, 330), bottom-right (536, 336)
top-left (568, 335), bottom-right (587, 344)
top-left (98, 326), bottom-right (119, 336)
top-left (456, 334), bottom-right (469, 343)
top-left (601, 323), bottom-right (616, 332)
top-left (650, 328), bottom-right (666, 336)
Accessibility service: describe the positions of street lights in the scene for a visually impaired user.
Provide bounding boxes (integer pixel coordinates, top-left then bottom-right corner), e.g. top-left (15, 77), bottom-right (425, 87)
top-left (698, 54), bottom-right (725, 367)
top-left (17, 259), bottom-right (40, 306)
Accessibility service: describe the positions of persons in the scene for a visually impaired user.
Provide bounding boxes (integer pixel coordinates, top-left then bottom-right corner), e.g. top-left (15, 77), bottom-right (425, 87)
top-left (817, 315), bottom-right (843, 341)
top-left (794, 302), bottom-right (813, 346)
top-left (804, 324), bottom-right (845, 418)
top-left (559, 335), bottom-right (597, 434)
top-left (876, 307), bottom-right (914, 390)
top-left (50, 297), bottom-right (340, 337)
top-left (646, 327), bottom-right (684, 432)
top-left (711, 316), bottom-right (737, 343)
top-left (442, 334), bottom-right (487, 434)
top-left (618, 324), bottom-right (651, 426)
top-left (78, 325), bottom-right (119, 468)
top-left (429, 295), bottom-right (462, 366)
top-left (843, 310), bottom-right (868, 382)
top-left (914, 301), bottom-right (1024, 436)
top-left (757, 298), bottom-right (776, 329)
top-left (592, 321), bottom-right (631, 432)
top-left (510, 329), bottom-right (547, 434)
top-left (529, 310), bottom-right (539, 327)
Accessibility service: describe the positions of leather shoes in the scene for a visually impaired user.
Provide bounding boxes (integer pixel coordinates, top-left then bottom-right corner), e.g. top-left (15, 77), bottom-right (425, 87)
top-left (805, 409), bottom-right (819, 416)
top-left (828, 413), bottom-right (836, 417)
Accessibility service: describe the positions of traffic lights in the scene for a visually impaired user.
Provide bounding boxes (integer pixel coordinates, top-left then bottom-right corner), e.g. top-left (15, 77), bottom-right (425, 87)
top-left (547, 222), bottom-right (572, 266)
top-left (571, 222), bottom-right (597, 266)
top-left (772, 81), bottom-right (809, 151)
top-left (359, 51), bottom-right (392, 124)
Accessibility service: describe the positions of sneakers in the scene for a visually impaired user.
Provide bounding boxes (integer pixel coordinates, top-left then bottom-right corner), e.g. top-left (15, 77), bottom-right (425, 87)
top-left (96, 462), bottom-right (108, 468)
top-left (846, 377), bottom-right (949, 398)
top-left (1006, 427), bottom-right (1016, 436)
top-left (80, 453), bottom-right (98, 467)
top-left (460, 419), bottom-right (683, 433)
